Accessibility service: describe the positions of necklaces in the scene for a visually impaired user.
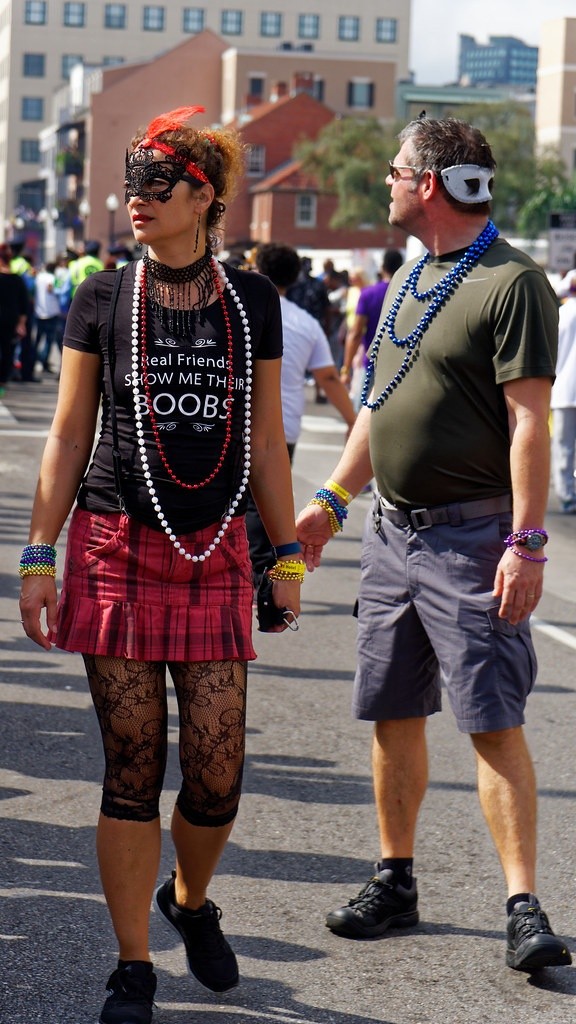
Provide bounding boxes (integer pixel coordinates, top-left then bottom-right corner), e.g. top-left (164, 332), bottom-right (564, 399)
top-left (131, 249), bottom-right (252, 562)
top-left (360, 221), bottom-right (499, 409)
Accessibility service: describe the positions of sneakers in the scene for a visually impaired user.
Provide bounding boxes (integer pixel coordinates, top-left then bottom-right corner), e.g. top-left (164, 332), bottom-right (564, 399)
top-left (326, 862), bottom-right (419, 937)
top-left (153, 869), bottom-right (239, 992)
top-left (96, 960), bottom-right (157, 1024)
top-left (503, 892), bottom-right (571, 969)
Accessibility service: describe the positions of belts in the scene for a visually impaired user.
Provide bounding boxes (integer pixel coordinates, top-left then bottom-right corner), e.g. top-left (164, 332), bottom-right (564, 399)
top-left (372, 488), bottom-right (512, 530)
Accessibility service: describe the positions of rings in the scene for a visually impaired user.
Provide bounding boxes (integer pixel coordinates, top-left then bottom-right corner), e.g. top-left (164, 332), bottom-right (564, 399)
top-left (527, 595), bottom-right (534, 597)
top-left (20, 620), bottom-right (24, 623)
top-left (308, 545), bottom-right (313, 548)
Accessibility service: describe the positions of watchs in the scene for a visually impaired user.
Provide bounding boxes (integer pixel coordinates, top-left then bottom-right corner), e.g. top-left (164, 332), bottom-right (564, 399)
top-left (519, 533), bottom-right (545, 549)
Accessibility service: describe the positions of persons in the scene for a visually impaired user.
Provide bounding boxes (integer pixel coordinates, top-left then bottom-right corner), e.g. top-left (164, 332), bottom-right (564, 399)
top-left (285, 248), bottom-right (405, 412)
top-left (292, 119), bottom-right (572, 971)
top-left (18, 125), bottom-right (307, 1024)
top-left (550, 269), bottom-right (575, 515)
top-left (0, 240), bottom-right (132, 387)
top-left (253, 243), bottom-right (356, 461)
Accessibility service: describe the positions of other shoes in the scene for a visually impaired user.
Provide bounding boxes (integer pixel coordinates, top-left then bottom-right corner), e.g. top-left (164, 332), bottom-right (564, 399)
top-left (564, 499), bottom-right (575, 514)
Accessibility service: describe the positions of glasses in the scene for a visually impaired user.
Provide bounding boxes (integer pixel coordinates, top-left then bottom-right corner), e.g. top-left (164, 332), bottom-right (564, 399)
top-left (389, 160), bottom-right (424, 180)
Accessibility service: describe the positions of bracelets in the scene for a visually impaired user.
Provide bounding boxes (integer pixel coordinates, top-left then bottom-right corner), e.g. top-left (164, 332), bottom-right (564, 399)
top-left (18, 543), bottom-right (57, 579)
top-left (324, 480), bottom-right (354, 504)
top-left (504, 529), bottom-right (549, 562)
top-left (268, 560), bottom-right (307, 584)
top-left (271, 541), bottom-right (301, 558)
top-left (308, 488), bottom-right (348, 537)
